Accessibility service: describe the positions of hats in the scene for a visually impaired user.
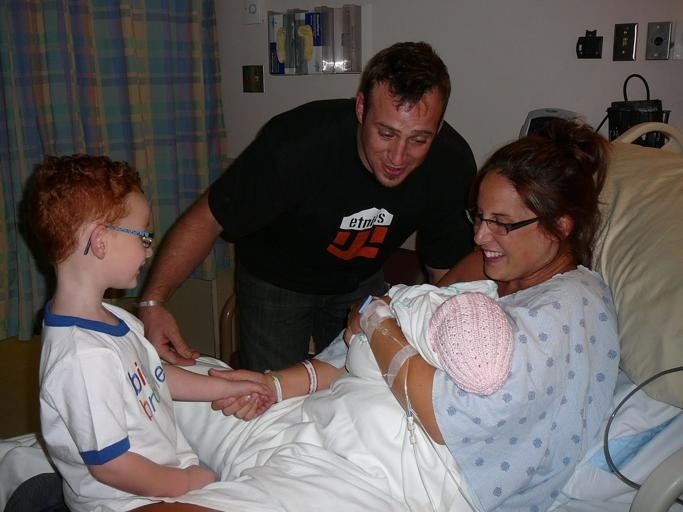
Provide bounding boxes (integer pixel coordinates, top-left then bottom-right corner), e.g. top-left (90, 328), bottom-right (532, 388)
top-left (429, 292), bottom-right (514, 395)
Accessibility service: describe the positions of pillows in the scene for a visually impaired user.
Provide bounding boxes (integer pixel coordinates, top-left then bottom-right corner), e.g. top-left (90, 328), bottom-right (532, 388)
top-left (577, 142), bottom-right (683, 409)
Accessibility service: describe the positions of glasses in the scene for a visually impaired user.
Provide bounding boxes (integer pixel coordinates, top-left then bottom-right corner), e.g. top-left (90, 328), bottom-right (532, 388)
top-left (464, 206), bottom-right (542, 236)
top-left (83, 223), bottom-right (154, 255)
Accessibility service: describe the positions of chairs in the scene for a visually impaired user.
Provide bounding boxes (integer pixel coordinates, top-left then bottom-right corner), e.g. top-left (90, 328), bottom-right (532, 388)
top-left (220, 248), bottom-right (427, 365)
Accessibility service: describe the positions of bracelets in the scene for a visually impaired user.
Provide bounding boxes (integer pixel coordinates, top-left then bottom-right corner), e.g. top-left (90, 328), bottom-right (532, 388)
top-left (263, 368), bottom-right (284, 404)
top-left (133, 298), bottom-right (165, 309)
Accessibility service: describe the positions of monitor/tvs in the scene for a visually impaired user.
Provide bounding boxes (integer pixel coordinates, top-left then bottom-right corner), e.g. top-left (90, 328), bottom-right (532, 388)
top-left (519, 107), bottom-right (577, 139)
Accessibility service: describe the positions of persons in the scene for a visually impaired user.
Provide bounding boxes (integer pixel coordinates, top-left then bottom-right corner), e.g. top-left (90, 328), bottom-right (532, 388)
top-left (207, 113), bottom-right (623, 512)
top-left (15, 149), bottom-right (274, 512)
top-left (128, 38), bottom-right (483, 377)
top-left (343, 274), bottom-right (517, 400)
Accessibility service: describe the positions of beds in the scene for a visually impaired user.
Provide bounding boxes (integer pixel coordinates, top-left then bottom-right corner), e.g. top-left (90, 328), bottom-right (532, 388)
top-left (0, 121), bottom-right (683, 512)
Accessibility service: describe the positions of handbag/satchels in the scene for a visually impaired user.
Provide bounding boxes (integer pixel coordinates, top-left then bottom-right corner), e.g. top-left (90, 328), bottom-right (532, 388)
top-left (594, 73), bottom-right (671, 149)
